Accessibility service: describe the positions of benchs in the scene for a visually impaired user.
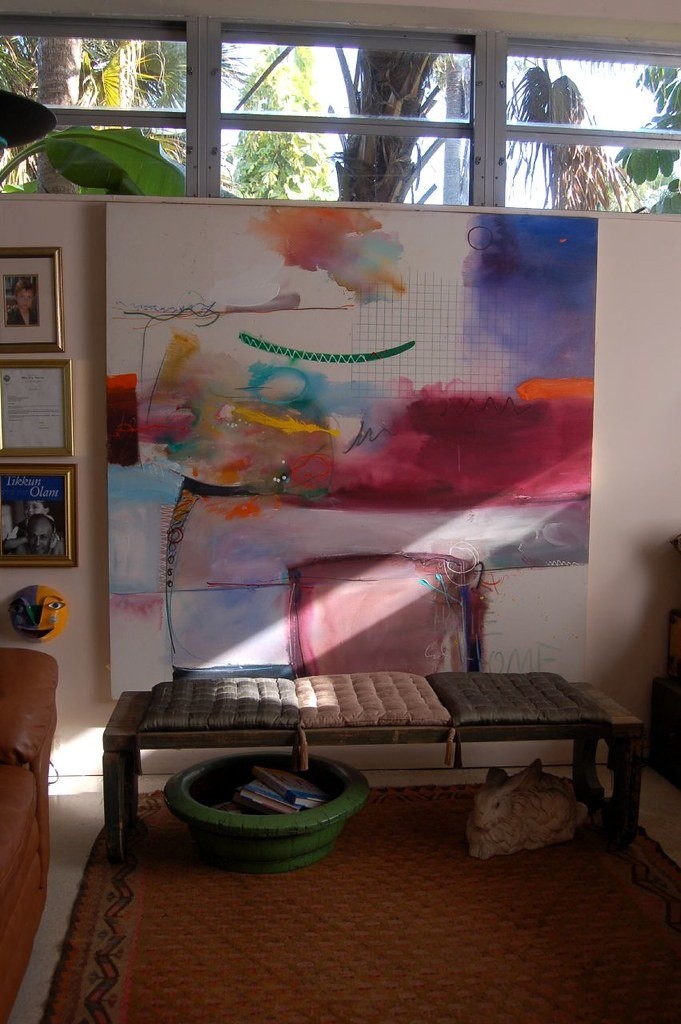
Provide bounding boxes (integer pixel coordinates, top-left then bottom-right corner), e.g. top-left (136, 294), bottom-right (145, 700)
top-left (102, 671), bottom-right (647, 865)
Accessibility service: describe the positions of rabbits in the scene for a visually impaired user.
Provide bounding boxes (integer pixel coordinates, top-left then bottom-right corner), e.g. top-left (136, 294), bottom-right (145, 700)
top-left (466, 758), bottom-right (588, 860)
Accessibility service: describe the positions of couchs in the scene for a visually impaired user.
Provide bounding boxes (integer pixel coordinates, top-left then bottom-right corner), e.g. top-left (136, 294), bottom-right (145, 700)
top-left (0, 647), bottom-right (60, 1024)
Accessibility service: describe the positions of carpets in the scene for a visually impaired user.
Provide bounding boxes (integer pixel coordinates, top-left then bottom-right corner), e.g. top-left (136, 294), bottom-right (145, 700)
top-left (42, 782), bottom-right (681, 1024)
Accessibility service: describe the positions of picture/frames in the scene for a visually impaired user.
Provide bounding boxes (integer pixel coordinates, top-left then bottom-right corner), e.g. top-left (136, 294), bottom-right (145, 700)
top-left (0, 246), bottom-right (65, 354)
top-left (0, 463), bottom-right (78, 567)
top-left (1, 358), bottom-right (75, 456)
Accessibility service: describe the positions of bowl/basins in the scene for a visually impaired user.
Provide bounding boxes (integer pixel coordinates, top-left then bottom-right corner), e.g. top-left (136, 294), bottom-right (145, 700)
top-left (165, 751), bottom-right (368, 873)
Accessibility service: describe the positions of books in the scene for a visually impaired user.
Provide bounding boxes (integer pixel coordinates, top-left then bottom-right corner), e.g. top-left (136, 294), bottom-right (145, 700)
top-left (233, 764), bottom-right (329, 814)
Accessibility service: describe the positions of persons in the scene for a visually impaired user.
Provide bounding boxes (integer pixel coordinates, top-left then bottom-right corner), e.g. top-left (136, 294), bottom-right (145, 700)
top-left (8, 276), bottom-right (36, 325)
top-left (5, 499), bottom-right (65, 556)
